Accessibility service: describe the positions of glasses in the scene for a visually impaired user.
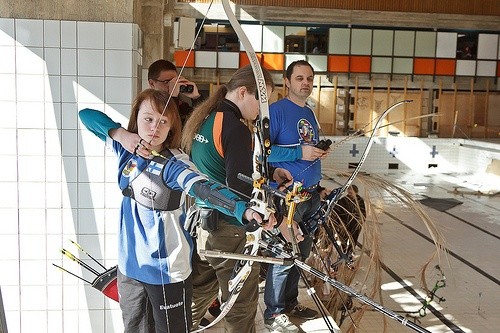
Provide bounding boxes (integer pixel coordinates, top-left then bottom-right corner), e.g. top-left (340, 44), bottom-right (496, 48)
top-left (156, 79), bottom-right (170, 84)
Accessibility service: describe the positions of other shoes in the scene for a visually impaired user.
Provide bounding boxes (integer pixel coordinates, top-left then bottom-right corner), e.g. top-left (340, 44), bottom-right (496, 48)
top-left (199, 319), bottom-right (211, 331)
top-left (208, 297), bottom-right (223, 318)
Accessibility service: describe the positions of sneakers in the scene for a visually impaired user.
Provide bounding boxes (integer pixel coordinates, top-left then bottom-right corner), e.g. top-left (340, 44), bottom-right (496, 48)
top-left (283, 301), bottom-right (319, 319)
top-left (263, 309), bottom-right (300, 332)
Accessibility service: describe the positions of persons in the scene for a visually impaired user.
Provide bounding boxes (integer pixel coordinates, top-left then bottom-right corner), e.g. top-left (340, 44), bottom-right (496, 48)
top-left (177, 61), bottom-right (275, 333)
top-left (79, 87), bottom-right (279, 333)
top-left (269, 60), bottom-right (332, 333)
top-left (148, 59), bottom-right (223, 328)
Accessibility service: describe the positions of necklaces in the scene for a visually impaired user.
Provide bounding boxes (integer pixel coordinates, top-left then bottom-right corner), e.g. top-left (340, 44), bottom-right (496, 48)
top-left (287, 95), bottom-right (291, 100)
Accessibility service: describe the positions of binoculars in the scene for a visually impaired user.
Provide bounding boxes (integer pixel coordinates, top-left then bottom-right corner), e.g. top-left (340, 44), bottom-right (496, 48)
top-left (315, 138), bottom-right (332, 152)
top-left (180, 81), bottom-right (194, 93)
